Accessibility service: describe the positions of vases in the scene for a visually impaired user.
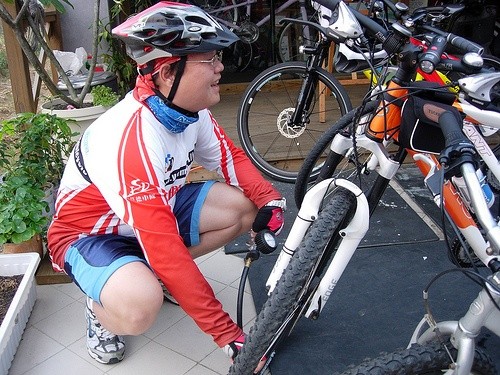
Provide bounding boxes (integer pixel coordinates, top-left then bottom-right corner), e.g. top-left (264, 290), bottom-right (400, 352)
top-left (0, 251), bottom-right (42, 375)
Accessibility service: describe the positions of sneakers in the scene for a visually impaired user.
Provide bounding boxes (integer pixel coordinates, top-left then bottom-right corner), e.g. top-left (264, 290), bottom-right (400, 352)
top-left (85, 295), bottom-right (125, 364)
top-left (157, 279), bottom-right (180, 306)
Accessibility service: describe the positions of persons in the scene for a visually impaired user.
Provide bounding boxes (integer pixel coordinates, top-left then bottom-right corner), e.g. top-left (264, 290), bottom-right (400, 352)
top-left (47, 0), bottom-right (285, 373)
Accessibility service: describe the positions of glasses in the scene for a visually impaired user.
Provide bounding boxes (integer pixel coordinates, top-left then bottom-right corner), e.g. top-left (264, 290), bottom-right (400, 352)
top-left (186, 50), bottom-right (223, 69)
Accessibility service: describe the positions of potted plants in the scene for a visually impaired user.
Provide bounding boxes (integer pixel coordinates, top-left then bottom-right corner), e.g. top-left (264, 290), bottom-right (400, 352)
top-left (0, 94), bottom-right (84, 258)
top-left (0, 0), bottom-right (121, 166)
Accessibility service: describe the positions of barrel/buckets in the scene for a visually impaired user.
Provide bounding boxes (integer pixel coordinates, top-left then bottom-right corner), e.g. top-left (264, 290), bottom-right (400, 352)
top-left (55, 71), bottom-right (120, 95)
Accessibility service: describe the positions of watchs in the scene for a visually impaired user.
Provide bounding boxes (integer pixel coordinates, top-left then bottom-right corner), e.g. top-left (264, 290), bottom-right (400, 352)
top-left (266, 198), bottom-right (287, 212)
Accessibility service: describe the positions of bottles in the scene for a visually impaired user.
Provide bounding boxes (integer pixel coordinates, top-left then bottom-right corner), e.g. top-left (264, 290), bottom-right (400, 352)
top-left (454, 165), bottom-right (495, 214)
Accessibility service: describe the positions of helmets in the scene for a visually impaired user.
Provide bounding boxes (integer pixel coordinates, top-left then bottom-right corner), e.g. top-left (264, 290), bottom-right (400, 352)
top-left (111, 0), bottom-right (241, 76)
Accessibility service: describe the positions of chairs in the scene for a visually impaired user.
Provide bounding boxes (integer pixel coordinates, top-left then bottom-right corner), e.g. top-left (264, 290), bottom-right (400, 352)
top-left (317, 0), bottom-right (371, 123)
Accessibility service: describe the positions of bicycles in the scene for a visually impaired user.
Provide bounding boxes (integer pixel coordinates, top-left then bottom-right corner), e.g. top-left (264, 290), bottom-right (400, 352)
top-left (203, 0), bottom-right (500, 375)
